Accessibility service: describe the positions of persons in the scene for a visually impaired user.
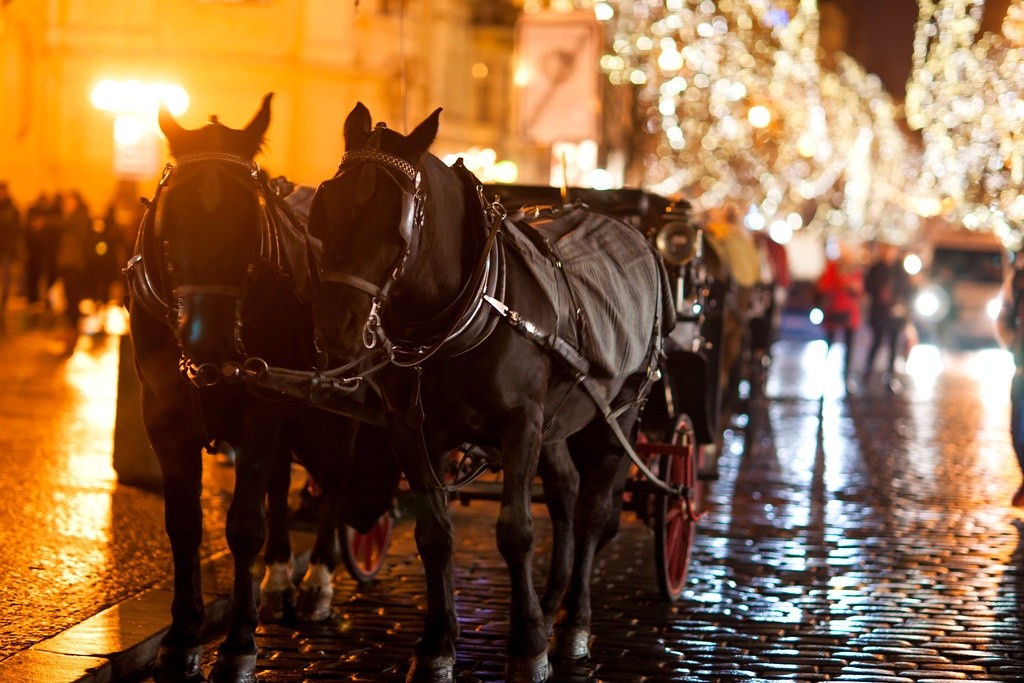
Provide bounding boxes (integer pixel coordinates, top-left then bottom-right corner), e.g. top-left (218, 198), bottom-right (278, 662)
top-left (817, 241), bottom-right (919, 396)
top-left (0, 176), bottom-right (147, 356)
top-left (993, 236), bottom-right (1023, 506)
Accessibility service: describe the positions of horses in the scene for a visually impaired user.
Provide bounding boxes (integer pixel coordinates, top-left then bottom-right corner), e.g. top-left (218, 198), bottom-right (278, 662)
top-left (115, 90), bottom-right (674, 682)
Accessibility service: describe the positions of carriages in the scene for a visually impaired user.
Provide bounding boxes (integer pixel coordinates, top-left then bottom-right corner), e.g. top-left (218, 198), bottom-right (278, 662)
top-left (124, 90), bottom-right (763, 683)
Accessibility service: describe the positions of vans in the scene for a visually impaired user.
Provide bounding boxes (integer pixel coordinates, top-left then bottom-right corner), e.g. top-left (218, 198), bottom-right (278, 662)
top-left (916, 232), bottom-right (1013, 346)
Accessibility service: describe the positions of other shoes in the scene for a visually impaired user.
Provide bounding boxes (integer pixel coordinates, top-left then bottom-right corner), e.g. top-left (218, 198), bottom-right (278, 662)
top-left (1012, 484), bottom-right (1024, 507)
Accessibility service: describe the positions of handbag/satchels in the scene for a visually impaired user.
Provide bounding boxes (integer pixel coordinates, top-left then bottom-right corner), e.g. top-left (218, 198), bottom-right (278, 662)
top-left (902, 323), bottom-right (917, 359)
